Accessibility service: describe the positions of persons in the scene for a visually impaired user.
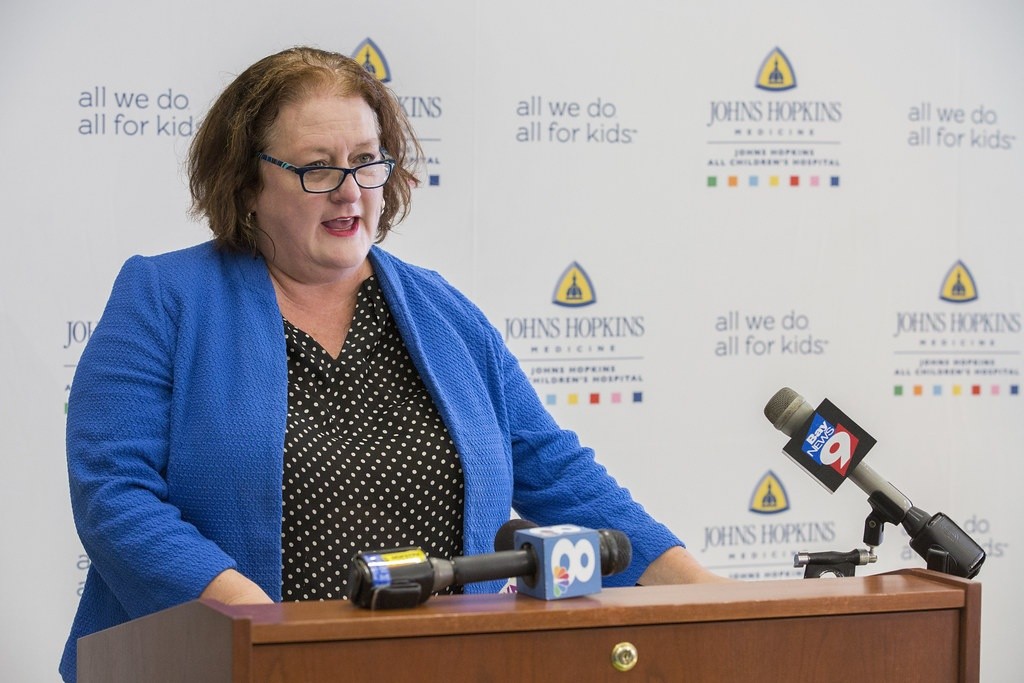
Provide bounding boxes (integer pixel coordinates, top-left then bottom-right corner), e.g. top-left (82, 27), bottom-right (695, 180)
top-left (59, 48), bottom-right (733, 683)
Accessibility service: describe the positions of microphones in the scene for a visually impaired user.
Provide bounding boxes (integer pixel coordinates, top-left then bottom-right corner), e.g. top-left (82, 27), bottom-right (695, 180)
top-left (346, 524), bottom-right (633, 612)
top-left (494, 519), bottom-right (540, 552)
top-left (764, 387), bottom-right (987, 580)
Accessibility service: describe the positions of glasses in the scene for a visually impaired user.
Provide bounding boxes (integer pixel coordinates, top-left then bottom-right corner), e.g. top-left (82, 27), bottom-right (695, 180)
top-left (256, 147), bottom-right (397, 194)
